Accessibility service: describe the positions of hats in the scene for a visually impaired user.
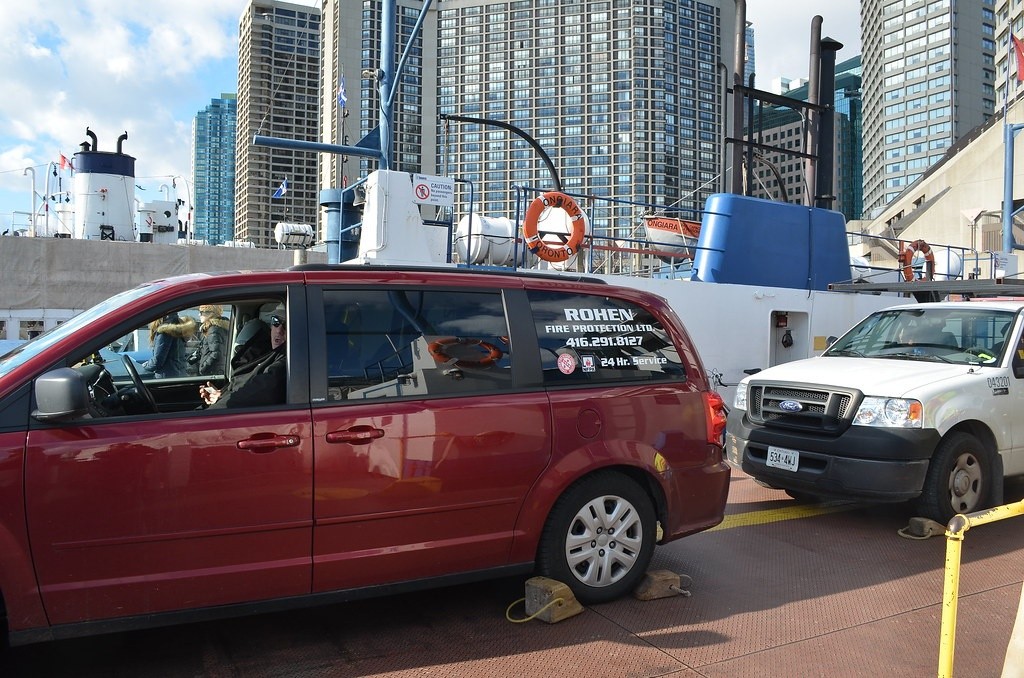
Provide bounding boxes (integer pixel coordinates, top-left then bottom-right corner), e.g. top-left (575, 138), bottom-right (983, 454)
top-left (199, 304), bottom-right (223, 315)
top-left (264, 302), bottom-right (285, 318)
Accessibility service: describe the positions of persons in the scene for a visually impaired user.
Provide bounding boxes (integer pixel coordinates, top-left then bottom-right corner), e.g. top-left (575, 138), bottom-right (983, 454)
top-left (186, 305), bottom-right (230, 376)
top-left (141, 311), bottom-right (196, 379)
top-left (978, 320), bottom-right (1024, 367)
top-left (197, 301), bottom-right (288, 411)
top-left (899, 325), bottom-right (920, 343)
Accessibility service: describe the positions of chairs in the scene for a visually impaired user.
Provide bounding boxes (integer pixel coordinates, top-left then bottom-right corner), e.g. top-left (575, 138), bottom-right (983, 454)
top-left (993, 321), bottom-right (1011, 353)
top-left (917, 319), bottom-right (957, 357)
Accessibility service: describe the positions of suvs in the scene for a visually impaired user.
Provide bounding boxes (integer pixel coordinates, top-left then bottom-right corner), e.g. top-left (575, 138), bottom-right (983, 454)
top-left (726, 297), bottom-right (1024, 529)
top-left (0, 262), bottom-right (732, 650)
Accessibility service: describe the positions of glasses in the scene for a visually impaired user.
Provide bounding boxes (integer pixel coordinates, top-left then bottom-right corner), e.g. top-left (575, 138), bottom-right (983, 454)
top-left (271, 316), bottom-right (286, 330)
top-left (199, 310), bottom-right (214, 317)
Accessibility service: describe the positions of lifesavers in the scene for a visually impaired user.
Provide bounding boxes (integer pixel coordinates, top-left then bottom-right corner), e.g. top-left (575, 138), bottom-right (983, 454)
top-left (427, 336), bottom-right (502, 367)
top-left (524, 193), bottom-right (584, 262)
top-left (903, 241), bottom-right (935, 280)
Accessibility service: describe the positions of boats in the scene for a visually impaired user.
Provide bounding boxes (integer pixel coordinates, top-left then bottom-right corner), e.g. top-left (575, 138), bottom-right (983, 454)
top-left (639, 214), bottom-right (702, 268)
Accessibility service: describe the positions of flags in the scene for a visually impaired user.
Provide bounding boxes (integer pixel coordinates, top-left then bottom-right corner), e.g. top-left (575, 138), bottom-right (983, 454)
top-left (338, 72), bottom-right (347, 107)
top-left (1011, 33), bottom-right (1024, 81)
top-left (60, 154), bottom-right (74, 171)
top-left (272, 178), bottom-right (288, 198)
